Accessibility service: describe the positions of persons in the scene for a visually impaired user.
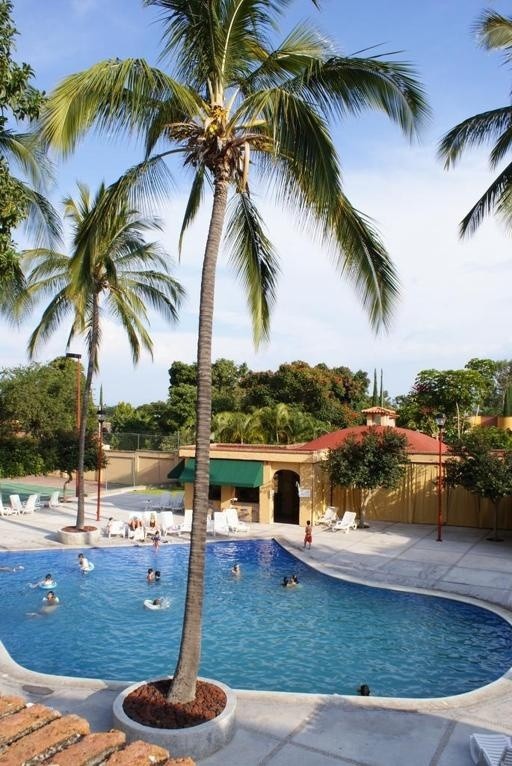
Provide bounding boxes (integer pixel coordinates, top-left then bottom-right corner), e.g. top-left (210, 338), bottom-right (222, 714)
top-left (41, 573), bottom-right (55, 586)
top-left (149, 510), bottom-right (159, 531)
top-left (77, 553), bottom-right (90, 575)
top-left (106, 516), bottom-right (113, 542)
top-left (356, 683), bottom-right (371, 696)
top-left (290, 575), bottom-right (299, 585)
top-left (303, 520), bottom-right (313, 550)
top-left (145, 566), bottom-right (154, 582)
top-left (123, 514), bottom-right (144, 531)
top-left (154, 570), bottom-right (160, 581)
top-left (46, 591), bottom-right (58, 605)
top-left (152, 598), bottom-right (161, 606)
top-left (150, 531), bottom-right (161, 551)
top-left (281, 576), bottom-right (291, 587)
top-left (231, 564), bottom-right (241, 574)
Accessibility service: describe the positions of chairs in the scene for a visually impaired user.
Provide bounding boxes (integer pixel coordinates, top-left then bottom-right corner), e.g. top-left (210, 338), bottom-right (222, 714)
top-left (319, 502), bottom-right (359, 534)
top-left (0, 481), bottom-right (62, 521)
top-left (104, 509), bottom-right (251, 544)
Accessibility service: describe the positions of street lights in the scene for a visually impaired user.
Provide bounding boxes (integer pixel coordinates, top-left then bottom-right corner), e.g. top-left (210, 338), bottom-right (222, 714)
top-left (436, 413), bottom-right (446, 542)
top-left (97, 410), bottom-right (105, 521)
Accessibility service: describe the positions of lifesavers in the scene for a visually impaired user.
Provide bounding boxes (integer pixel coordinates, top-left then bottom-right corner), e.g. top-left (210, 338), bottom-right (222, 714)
top-left (39, 582), bottom-right (57, 589)
top-left (144, 600), bottom-right (169, 610)
top-left (82, 561), bottom-right (94, 571)
top-left (151, 537), bottom-right (161, 540)
